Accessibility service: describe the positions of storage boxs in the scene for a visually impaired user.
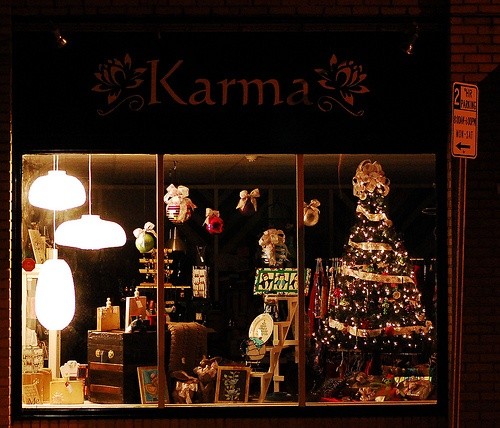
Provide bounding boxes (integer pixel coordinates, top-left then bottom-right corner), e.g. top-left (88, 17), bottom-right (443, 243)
top-left (49, 377), bottom-right (85, 404)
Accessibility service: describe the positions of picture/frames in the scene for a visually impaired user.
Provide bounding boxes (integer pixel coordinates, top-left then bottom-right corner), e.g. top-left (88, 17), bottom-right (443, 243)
top-left (214, 366), bottom-right (251, 404)
top-left (136, 367), bottom-right (169, 404)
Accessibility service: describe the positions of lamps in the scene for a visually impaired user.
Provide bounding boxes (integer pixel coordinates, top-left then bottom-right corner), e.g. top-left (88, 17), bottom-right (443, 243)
top-left (29, 153), bottom-right (128, 332)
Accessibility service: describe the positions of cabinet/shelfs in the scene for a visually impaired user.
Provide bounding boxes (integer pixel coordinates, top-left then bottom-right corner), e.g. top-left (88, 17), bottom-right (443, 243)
top-left (251, 295), bottom-right (302, 402)
top-left (86, 329), bottom-right (167, 405)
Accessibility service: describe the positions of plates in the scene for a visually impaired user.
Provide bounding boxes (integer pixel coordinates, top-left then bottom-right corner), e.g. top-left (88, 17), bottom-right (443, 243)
top-left (248, 313), bottom-right (274, 345)
